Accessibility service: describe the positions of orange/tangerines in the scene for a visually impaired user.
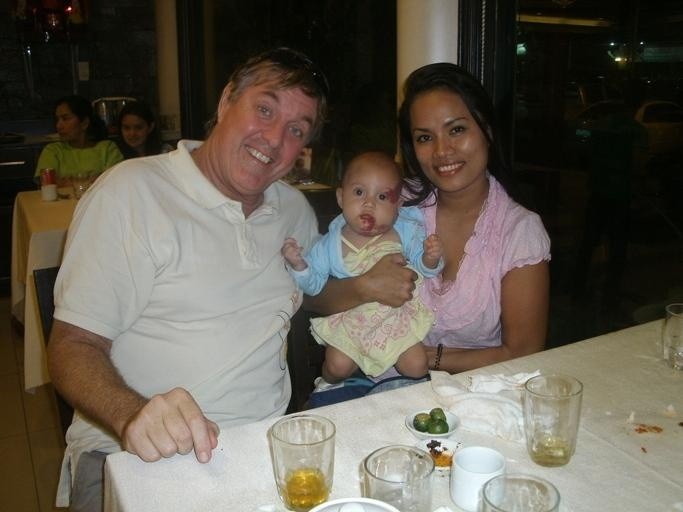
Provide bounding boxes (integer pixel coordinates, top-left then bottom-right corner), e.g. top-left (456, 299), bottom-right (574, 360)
top-left (414, 408), bottom-right (449, 433)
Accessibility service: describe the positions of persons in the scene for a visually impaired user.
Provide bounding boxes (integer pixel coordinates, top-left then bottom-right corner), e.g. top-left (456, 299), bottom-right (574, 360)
top-left (565, 79), bottom-right (650, 299)
top-left (32, 95), bottom-right (125, 190)
top-left (46, 47), bottom-right (319, 512)
top-left (282, 152), bottom-right (445, 385)
top-left (302, 63), bottom-right (552, 374)
top-left (117, 101), bottom-right (175, 160)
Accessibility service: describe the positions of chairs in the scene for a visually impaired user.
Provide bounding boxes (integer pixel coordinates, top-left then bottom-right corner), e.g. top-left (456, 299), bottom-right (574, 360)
top-left (33, 269), bottom-right (306, 444)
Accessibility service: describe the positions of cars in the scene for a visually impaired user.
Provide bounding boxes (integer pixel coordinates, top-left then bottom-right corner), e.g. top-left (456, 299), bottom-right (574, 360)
top-left (636, 97), bottom-right (680, 151)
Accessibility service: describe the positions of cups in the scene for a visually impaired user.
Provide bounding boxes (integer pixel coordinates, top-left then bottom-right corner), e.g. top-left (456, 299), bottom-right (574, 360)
top-left (451, 446), bottom-right (513, 511)
top-left (365, 444), bottom-right (436, 512)
top-left (42, 184), bottom-right (59, 201)
top-left (484, 474), bottom-right (561, 512)
top-left (525, 374), bottom-right (584, 467)
top-left (270, 416), bottom-right (337, 512)
top-left (663, 303), bottom-right (683, 369)
top-left (71, 171), bottom-right (90, 200)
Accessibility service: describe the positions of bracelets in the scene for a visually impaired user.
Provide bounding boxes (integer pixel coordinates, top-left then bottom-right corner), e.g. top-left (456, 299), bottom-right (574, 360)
top-left (434, 343), bottom-right (444, 371)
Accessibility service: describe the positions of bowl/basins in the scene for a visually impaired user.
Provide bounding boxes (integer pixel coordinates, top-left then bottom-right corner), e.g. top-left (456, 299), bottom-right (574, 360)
top-left (304, 497), bottom-right (399, 512)
top-left (405, 410), bottom-right (469, 436)
